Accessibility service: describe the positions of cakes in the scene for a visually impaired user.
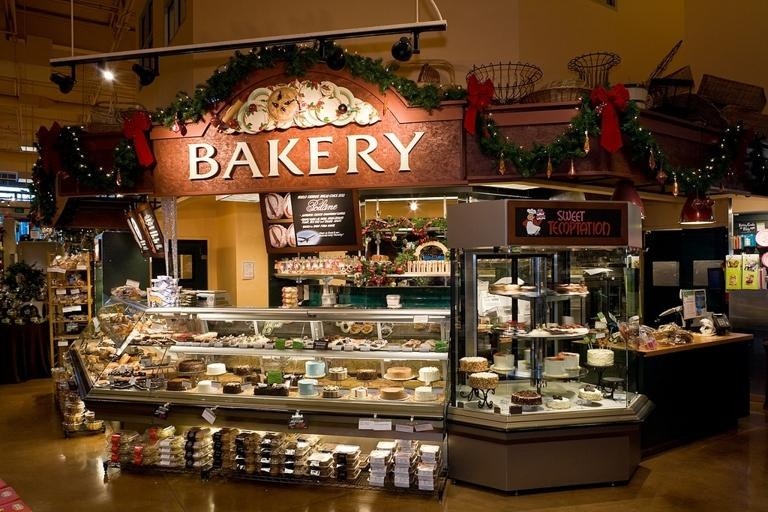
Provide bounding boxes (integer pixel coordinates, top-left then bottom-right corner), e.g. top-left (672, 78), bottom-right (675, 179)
top-left (97, 304), bottom-right (147, 338)
top-left (48, 254), bottom-right (91, 338)
top-left (53, 367), bottom-right (103, 431)
top-left (336, 322), bottom-right (375, 334)
top-left (414, 323), bottom-right (441, 334)
top-left (329, 338), bottom-right (391, 350)
top-left (194, 335), bottom-right (275, 350)
top-left (381, 323), bottom-right (394, 337)
top-left (457, 356), bottom-right (500, 389)
top-left (545, 395), bottom-right (570, 408)
top-left (115, 284), bottom-right (143, 301)
top-left (263, 321), bottom-right (283, 335)
top-left (511, 389), bottom-right (544, 404)
top-left (578, 385), bottom-right (602, 401)
top-left (110, 350), bottom-right (165, 388)
top-left (134, 319), bottom-right (194, 348)
top-left (77, 330), bottom-right (118, 376)
top-left (350, 367), bottom-right (441, 400)
top-left (256, 435), bottom-right (368, 484)
top-left (588, 350), bottom-right (614, 365)
top-left (276, 338), bottom-right (328, 349)
top-left (368, 439), bottom-right (443, 492)
top-left (255, 361), bottom-right (349, 399)
top-left (486, 281), bottom-right (589, 375)
top-left (166, 360), bottom-right (253, 393)
top-left (106, 424), bottom-right (185, 468)
top-left (185, 426), bottom-right (258, 472)
top-left (401, 340), bottom-right (446, 351)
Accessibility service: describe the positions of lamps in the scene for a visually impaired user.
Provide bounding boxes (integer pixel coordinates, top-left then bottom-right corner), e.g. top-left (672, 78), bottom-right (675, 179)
top-left (608, 179), bottom-right (648, 219)
top-left (389, 30), bottom-right (422, 59)
top-left (131, 56), bottom-right (161, 88)
top-left (47, 65), bottom-right (81, 96)
top-left (677, 192), bottom-right (717, 228)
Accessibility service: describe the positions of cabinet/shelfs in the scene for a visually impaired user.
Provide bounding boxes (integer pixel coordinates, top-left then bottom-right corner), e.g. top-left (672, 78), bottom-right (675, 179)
top-left (104, 327), bottom-right (447, 409)
top-left (474, 256), bottom-right (589, 390)
top-left (274, 257), bottom-right (451, 308)
top-left (48, 251), bottom-right (93, 369)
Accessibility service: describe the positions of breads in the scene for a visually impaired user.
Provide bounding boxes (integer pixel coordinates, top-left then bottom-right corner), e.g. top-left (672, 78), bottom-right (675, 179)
top-left (615, 323), bottom-right (692, 347)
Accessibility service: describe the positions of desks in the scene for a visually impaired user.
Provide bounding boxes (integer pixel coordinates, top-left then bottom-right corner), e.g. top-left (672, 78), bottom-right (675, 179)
top-left (568, 325), bottom-right (754, 458)
